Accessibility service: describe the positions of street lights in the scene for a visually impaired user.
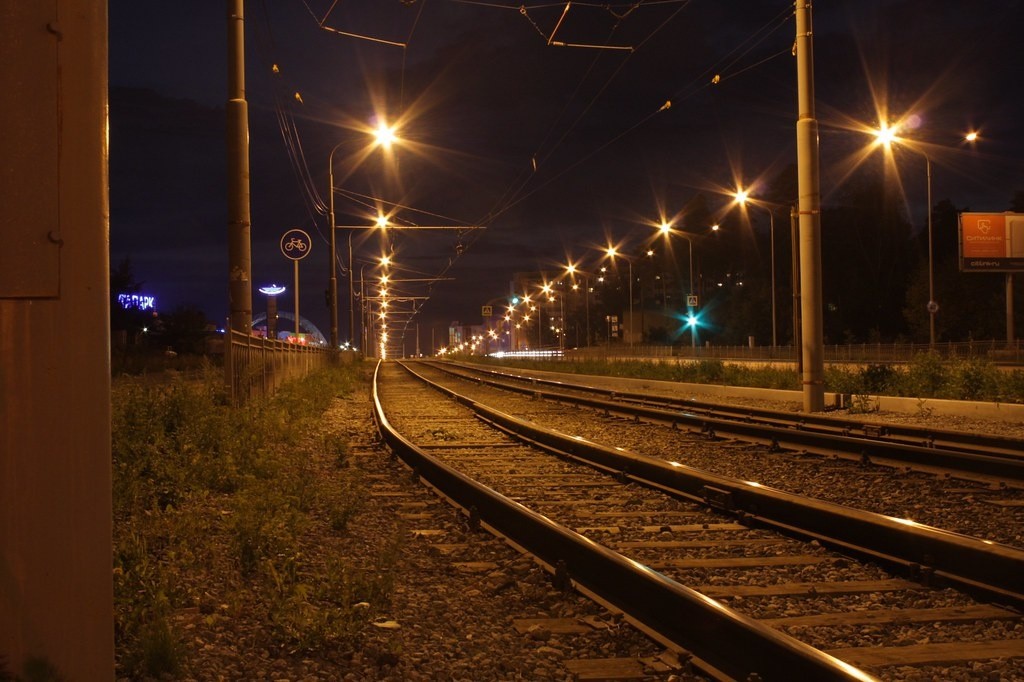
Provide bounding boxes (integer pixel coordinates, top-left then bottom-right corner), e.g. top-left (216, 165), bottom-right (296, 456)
top-left (360, 258), bottom-right (389, 357)
top-left (736, 194), bottom-right (776, 351)
top-left (661, 224), bottom-right (695, 348)
top-left (569, 266), bottom-right (590, 348)
top-left (608, 249), bottom-right (633, 348)
top-left (872, 130), bottom-right (934, 349)
top-left (543, 287), bottom-right (564, 349)
top-left (348, 219), bottom-right (387, 339)
top-left (329, 129), bottom-right (392, 346)
top-left (523, 297), bottom-right (543, 348)
